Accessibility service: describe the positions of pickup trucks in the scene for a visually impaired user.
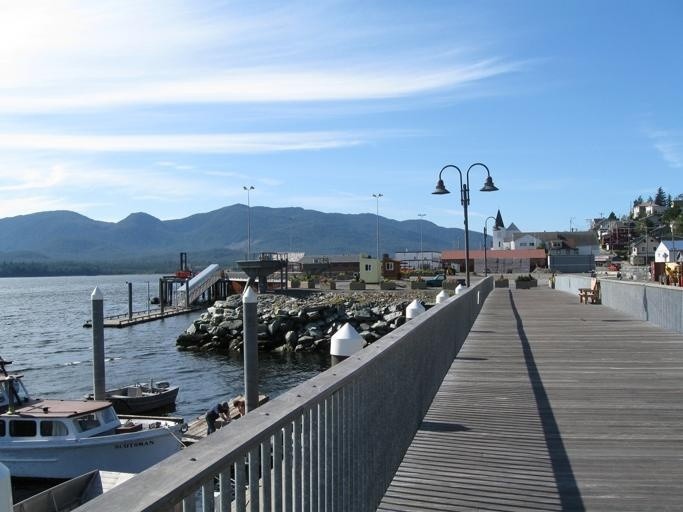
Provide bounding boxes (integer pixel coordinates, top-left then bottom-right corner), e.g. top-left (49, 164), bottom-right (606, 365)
top-left (426, 274), bottom-right (465, 287)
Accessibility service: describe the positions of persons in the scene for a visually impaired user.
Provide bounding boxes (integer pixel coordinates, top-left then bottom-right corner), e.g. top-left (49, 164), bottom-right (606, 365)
top-left (232, 400), bottom-right (244, 416)
top-left (205, 401), bottom-right (230, 435)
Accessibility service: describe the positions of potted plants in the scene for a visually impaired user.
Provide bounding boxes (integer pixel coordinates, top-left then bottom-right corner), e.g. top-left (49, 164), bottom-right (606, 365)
top-left (515, 274), bottom-right (537, 289)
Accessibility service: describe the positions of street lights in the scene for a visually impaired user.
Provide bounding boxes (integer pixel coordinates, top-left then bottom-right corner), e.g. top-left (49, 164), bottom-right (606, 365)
top-left (483, 216), bottom-right (500, 276)
top-left (417, 213), bottom-right (425, 275)
top-left (431, 163), bottom-right (499, 288)
top-left (372, 193), bottom-right (383, 285)
top-left (243, 185), bottom-right (254, 260)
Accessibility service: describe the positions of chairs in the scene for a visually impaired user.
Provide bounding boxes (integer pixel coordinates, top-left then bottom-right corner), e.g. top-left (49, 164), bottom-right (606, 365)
top-left (578, 278), bottom-right (600, 304)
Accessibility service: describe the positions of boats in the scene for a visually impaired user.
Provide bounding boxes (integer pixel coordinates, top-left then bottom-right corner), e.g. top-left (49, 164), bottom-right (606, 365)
top-left (0, 357), bottom-right (188, 480)
top-left (83, 378), bottom-right (178, 415)
